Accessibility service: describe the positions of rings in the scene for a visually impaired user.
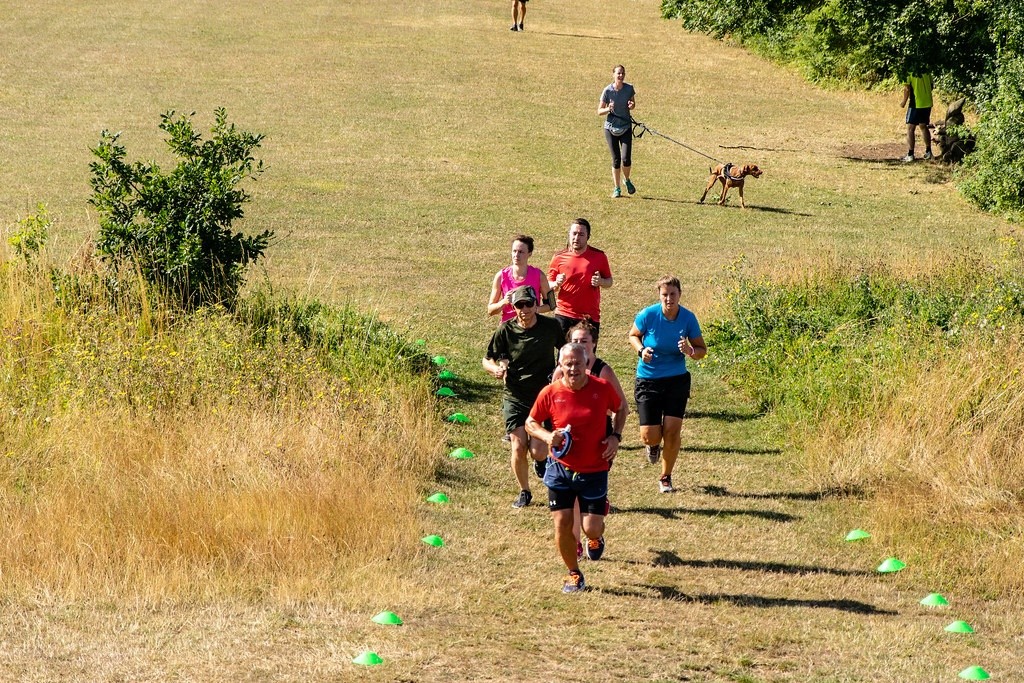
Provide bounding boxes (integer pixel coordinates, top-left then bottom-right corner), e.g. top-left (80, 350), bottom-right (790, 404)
top-left (613, 452), bottom-right (616, 454)
top-left (646, 357), bottom-right (648, 359)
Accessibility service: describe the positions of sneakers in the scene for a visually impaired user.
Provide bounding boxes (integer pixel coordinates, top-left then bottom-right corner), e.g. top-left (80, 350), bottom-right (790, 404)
top-left (659, 474), bottom-right (674, 493)
top-left (623, 178), bottom-right (635, 194)
top-left (646, 445), bottom-right (660, 464)
top-left (613, 187), bottom-right (621, 198)
top-left (533, 458), bottom-right (548, 478)
top-left (563, 570), bottom-right (585, 594)
top-left (512, 490), bottom-right (532, 508)
top-left (586, 536), bottom-right (605, 561)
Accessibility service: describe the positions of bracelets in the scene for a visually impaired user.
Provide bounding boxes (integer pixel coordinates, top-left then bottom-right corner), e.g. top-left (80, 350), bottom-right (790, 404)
top-left (689, 346), bottom-right (695, 355)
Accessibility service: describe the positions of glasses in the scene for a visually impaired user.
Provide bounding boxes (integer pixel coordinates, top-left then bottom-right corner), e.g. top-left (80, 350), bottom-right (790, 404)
top-left (514, 300), bottom-right (534, 309)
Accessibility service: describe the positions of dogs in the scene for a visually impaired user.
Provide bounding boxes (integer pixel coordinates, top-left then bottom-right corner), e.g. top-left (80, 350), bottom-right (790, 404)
top-left (700, 164), bottom-right (763, 208)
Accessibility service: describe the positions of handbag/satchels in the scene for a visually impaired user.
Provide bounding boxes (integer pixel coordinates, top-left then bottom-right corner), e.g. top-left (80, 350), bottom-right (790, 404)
top-left (609, 127), bottom-right (628, 137)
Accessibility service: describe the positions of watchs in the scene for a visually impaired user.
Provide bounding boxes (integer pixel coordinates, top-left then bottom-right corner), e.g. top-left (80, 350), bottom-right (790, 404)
top-left (638, 348), bottom-right (644, 358)
top-left (613, 432), bottom-right (622, 442)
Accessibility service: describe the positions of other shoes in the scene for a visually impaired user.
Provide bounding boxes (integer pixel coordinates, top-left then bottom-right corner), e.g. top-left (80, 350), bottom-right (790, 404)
top-left (604, 498), bottom-right (609, 516)
top-left (905, 154), bottom-right (914, 162)
top-left (576, 542), bottom-right (583, 556)
top-left (518, 23), bottom-right (523, 30)
top-left (923, 151), bottom-right (933, 160)
top-left (510, 24), bottom-right (518, 31)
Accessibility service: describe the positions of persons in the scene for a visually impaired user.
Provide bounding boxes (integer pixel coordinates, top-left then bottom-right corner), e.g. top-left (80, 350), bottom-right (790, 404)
top-left (487, 235), bottom-right (557, 324)
top-left (551, 314), bottom-right (630, 554)
top-left (598, 64), bottom-right (636, 197)
top-left (547, 218), bottom-right (613, 365)
top-left (900, 64), bottom-right (934, 162)
top-left (510, 0), bottom-right (529, 31)
top-left (524, 341), bottom-right (626, 593)
top-left (629, 274), bottom-right (707, 493)
top-left (482, 285), bottom-right (568, 508)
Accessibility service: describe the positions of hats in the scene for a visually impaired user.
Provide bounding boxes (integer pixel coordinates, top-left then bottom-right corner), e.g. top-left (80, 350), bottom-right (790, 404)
top-left (512, 285), bottom-right (536, 304)
